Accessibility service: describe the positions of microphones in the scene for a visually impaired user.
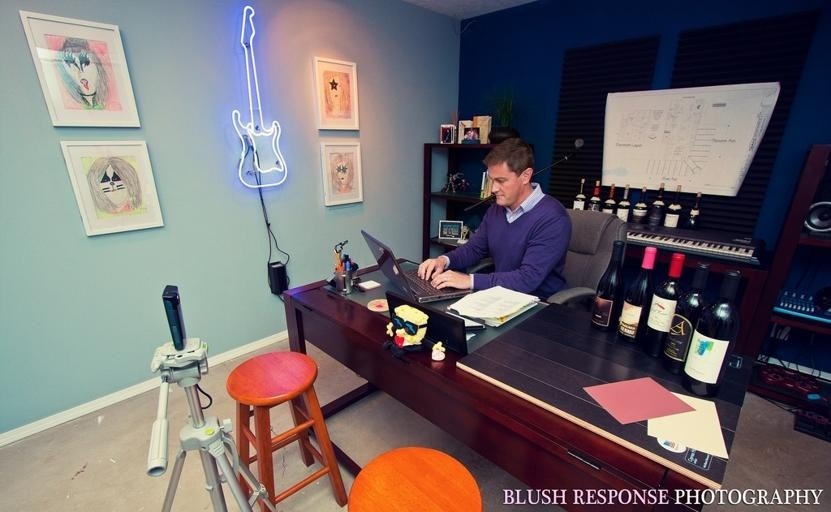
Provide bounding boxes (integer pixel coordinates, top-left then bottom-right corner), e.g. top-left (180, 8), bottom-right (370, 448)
top-left (564, 138), bottom-right (585, 160)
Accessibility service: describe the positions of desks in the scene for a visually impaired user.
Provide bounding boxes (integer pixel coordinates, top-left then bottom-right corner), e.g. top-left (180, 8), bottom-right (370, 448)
top-left (282, 258), bottom-right (747, 512)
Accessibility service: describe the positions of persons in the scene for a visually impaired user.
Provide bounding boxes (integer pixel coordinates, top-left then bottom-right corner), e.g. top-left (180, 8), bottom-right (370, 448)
top-left (385, 304), bottom-right (429, 346)
top-left (416, 137), bottom-right (573, 301)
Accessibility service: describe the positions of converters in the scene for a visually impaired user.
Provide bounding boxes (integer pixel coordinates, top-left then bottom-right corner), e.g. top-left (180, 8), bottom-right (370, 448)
top-left (268, 261), bottom-right (288, 295)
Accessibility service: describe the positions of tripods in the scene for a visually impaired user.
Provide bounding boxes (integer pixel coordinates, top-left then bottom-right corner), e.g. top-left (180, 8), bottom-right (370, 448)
top-left (146, 338), bottom-right (277, 512)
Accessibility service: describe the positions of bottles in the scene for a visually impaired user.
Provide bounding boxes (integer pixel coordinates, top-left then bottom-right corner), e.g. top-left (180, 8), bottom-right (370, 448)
top-left (664, 184), bottom-right (684, 227)
top-left (633, 186), bottom-right (647, 223)
top-left (616, 184), bottom-right (631, 222)
top-left (682, 268), bottom-right (744, 395)
top-left (648, 183), bottom-right (665, 224)
top-left (602, 184), bottom-right (616, 213)
top-left (573, 178), bottom-right (586, 210)
top-left (590, 240), bottom-right (629, 327)
top-left (615, 247), bottom-right (658, 335)
top-left (588, 180), bottom-right (601, 211)
top-left (638, 253), bottom-right (686, 351)
top-left (686, 191), bottom-right (702, 229)
top-left (662, 263), bottom-right (712, 367)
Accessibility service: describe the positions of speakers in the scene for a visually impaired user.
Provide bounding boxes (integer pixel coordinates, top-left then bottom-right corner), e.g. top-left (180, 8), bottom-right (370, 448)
top-left (788, 143), bottom-right (831, 244)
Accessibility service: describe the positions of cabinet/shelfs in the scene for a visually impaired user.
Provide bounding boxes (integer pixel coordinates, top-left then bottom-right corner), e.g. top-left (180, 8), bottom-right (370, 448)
top-left (624, 244), bottom-right (768, 356)
top-left (422, 143), bottom-right (535, 268)
top-left (739, 144), bottom-right (831, 418)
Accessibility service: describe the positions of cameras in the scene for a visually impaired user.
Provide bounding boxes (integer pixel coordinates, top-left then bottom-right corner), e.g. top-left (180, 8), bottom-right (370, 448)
top-left (162, 285), bottom-right (191, 350)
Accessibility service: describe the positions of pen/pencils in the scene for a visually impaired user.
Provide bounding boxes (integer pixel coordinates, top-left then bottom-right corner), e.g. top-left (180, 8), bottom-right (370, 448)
top-left (333, 249), bottom-right (354, 272)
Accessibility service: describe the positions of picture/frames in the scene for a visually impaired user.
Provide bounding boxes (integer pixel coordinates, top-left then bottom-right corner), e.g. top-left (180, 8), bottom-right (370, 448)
top-left (314, 56), bottom-right (359, 131)
top-left (440, 124), bottom-right (455, 144)
top-left (321, 142), bottom-right (363, 206)
top-left (458, 120), bottom-right (487, 145)
top-left (438, 220), bottom-right (463, 240)
top-left (61, 141), bottom-right (164, 237)
top-left (19, 9), bottom-right (141, 127)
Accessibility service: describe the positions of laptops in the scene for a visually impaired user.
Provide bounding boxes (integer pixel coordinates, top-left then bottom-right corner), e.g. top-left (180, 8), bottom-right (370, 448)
top-left (361, 229), bottom-right (472, 304)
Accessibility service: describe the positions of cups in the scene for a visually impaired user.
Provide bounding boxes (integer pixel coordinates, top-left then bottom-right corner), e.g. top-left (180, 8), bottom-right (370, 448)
top-left (334, 267), bottom-right (357, 294)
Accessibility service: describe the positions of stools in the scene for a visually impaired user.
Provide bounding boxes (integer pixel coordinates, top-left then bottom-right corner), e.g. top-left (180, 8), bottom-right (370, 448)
top-left (225, 352), bottom-right (348, 512)
top-left (348, 446), bottom-right (483, 512)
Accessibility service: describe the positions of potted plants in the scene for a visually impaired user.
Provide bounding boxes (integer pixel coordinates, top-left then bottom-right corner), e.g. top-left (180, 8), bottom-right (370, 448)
top-left (488, 95), bottom-right (520, 143)
